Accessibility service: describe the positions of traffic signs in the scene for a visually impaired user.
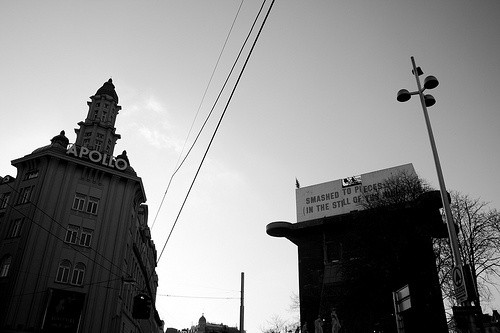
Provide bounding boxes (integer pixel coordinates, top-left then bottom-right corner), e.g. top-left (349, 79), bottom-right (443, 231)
top-left (450, 264), bottom-right (468, 304)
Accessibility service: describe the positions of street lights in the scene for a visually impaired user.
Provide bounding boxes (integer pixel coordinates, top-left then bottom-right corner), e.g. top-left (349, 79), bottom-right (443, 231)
top-left (396, 55), bottom-right (477, 333)
